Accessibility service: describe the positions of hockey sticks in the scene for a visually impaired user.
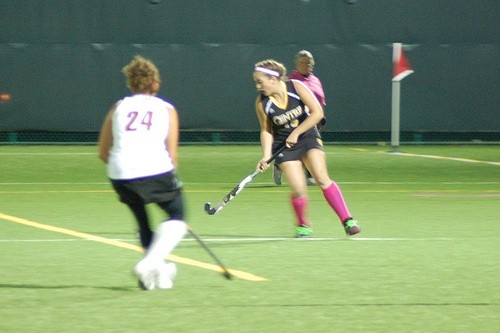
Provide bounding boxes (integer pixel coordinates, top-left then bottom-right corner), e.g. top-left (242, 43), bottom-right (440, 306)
top-left (187, 230), bottom-right (231, 279)
top-left (205, 145), bottom-right (286, 215)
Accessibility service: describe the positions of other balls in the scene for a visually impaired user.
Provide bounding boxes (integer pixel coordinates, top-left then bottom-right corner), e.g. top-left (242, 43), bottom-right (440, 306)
top-left (136, 232), bottom-right (141, 240)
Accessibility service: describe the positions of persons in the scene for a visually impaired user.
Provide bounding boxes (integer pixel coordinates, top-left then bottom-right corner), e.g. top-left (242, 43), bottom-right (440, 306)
top-left (273, 50), bottom-right (330, 186)
top-left (98, 55), bottom-right (189, 291)
top-left (252, 60), bottom-right (361, 237)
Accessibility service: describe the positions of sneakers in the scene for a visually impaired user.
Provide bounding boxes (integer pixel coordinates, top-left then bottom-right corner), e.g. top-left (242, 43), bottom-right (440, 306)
top-left (158, 263), bottom-right (176, 289)
top-left (296, 225), bottom-right (312, 237)
top-left (344, 218), bottom-right (360, 235)
top-left (135, 259), bottom-right (155, 290)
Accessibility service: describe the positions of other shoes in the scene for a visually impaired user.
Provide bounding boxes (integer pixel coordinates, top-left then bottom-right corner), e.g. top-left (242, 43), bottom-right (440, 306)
top-left (307, 178), bottom-right (317, 185)
top-left (274, 164), bottom-right (282, 185)
